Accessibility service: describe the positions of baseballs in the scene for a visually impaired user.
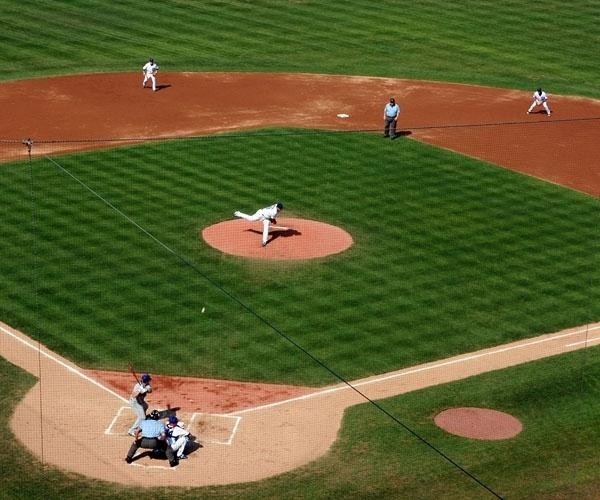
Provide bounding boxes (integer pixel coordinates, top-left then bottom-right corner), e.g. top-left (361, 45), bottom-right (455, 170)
top-left (201, 307), bottom-right (205, 314)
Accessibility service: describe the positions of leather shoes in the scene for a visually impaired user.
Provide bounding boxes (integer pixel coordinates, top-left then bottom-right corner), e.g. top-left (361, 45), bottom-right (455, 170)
top-left (170, 461), bottom-right (178, 466)
top-left (125, 457), bottom-right (131, 463)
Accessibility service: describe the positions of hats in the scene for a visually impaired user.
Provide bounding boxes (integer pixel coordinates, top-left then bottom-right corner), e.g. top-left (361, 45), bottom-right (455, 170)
top-left (537, 88), bottom-right (542, 92)
top-left (390, 99), bottom-right (395, 103)
top-left (150, 59), bottom-right (154, 62)
top-left (277, 203), bottom-right (283, 210)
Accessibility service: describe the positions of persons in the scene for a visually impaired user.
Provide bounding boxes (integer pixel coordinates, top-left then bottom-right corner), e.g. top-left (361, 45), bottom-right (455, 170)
top-left (383, 98), bottom-right (400, 140)
top-left (125, 410), bottom-right (179, 467)
top-left (526, 88), bottom-right (551, 117)
top-left (234, 203), bottom-right (283, 247)
top-left (128, 375), bottom-right (152, 438)
top-left (142, 59), bottom-right (159, 92)
top-left (164, 416), bottom-right (192, 459)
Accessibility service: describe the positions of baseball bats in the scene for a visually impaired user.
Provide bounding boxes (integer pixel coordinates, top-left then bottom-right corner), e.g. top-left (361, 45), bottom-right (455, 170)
top-left (127, 362), bottom-right (139, 384)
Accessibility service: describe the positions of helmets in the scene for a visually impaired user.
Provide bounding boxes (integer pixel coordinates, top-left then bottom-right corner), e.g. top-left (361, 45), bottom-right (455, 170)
top-left (141, 374), bottom-right (150, 382)
top-left (151, 410), bottom-right (160, 418)
top-left (170, 417), bottom-right (178, 423)
